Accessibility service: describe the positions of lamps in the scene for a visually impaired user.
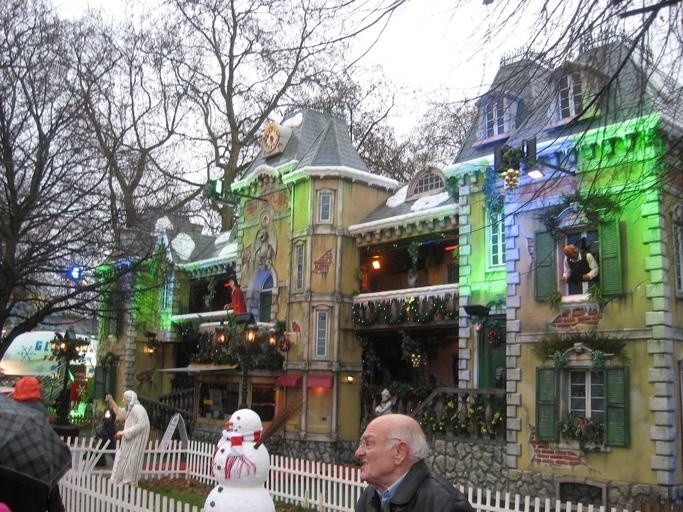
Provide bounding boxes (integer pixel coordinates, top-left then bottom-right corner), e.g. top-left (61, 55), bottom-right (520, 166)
top-left (243, 322), bottom-right (258, 344)
top-left (215, 320), bottom-right (228, 343)
top-left (527, 158), bottom-right (575, 180)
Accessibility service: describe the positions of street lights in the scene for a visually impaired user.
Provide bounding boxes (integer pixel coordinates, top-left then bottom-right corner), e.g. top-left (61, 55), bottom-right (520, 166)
top-left (213, 312), bottom-right (276, 409)
top-left (49, 330), bottom-right (89, 425)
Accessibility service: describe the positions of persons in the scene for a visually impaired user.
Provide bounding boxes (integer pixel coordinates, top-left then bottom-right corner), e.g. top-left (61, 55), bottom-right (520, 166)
top-left (0, 374), bottom-right (72, 511)
top-left (102, 389), bottom-right (150, 490)
top-left (222, 274), bottom-right (246, 315)
top-left (374, 388), bottom-right (392, 416)
top-left (351, 412), bottom-right (478, 512)
top-left (559, 244), bottom-right (598, 295)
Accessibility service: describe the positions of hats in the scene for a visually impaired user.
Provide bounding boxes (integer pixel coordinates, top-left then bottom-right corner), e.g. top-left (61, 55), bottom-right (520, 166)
top-left (13, 376), bottom-right (42, 401)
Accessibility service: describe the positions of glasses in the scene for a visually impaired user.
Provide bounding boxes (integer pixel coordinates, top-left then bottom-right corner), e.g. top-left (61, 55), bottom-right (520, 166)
top-left (358, 438), bottom-right (401, 451)
